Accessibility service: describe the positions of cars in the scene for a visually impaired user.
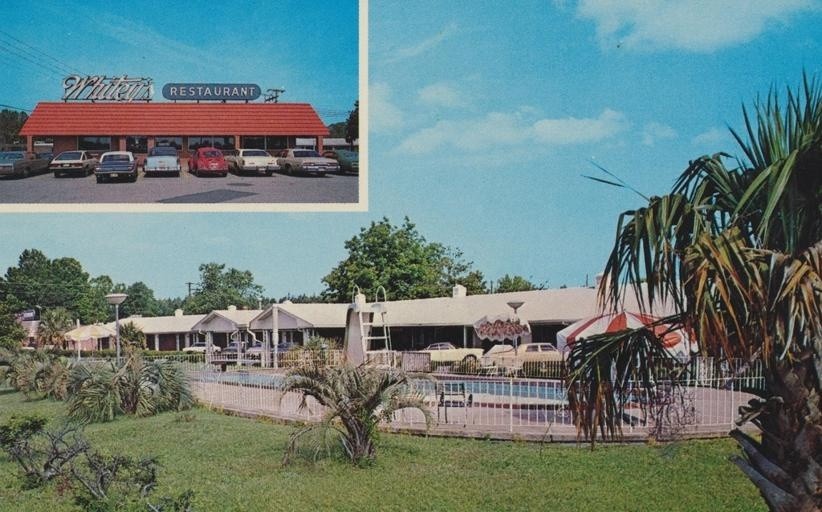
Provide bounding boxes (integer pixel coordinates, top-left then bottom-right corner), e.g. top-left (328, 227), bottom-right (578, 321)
top-left (419, 342), bottom-right (561, 367)
top-left (183, 341), bottom-right (294, 360)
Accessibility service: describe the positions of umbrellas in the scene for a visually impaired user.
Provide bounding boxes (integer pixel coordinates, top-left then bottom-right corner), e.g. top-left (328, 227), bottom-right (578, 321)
top-left (556, 308), bottom-right (683, 355)
top-left (473, 312), bottom-right (531, 375)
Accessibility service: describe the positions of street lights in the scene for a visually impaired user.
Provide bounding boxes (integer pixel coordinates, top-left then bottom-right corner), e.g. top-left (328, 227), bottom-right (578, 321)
top-left (104, 294), bottom-right (127, 365)
top-left (36, 305), bottom-right (42, 321)
top-left (506, 301), bottom-right (526, 357)
